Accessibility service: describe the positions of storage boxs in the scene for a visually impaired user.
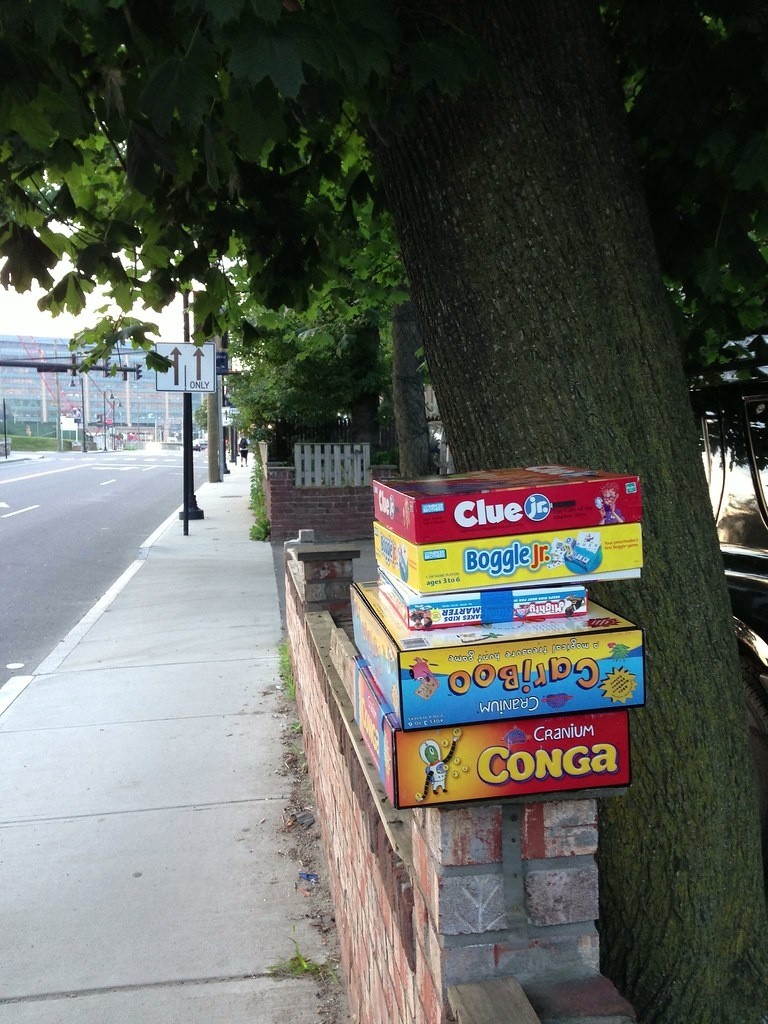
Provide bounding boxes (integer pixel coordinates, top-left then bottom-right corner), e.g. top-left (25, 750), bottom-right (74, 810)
top-left (376, 566), bottom-right (587, 631)
top-left (351, 653), bottom-right (631, 808)
top-left (348, 584), bottom-right (646, 732)
top-left (371, 463), bottom-right (640, 546)
top-left (363, 522), bottom-right (642, 597)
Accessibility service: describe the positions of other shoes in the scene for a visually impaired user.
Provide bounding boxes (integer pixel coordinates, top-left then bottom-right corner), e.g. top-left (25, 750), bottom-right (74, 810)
top-left (246, 465), bottom-right (248, 466)
top-left (241, 464), bottom-right (243, 467)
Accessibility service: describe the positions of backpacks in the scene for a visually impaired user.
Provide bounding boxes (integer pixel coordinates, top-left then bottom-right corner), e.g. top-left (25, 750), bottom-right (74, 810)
top-left (239, 437), bottom-right (247, 447)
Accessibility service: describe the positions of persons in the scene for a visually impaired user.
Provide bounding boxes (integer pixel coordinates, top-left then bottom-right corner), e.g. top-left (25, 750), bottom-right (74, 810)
top-left (238, 434), bottom-right (251, 467)
